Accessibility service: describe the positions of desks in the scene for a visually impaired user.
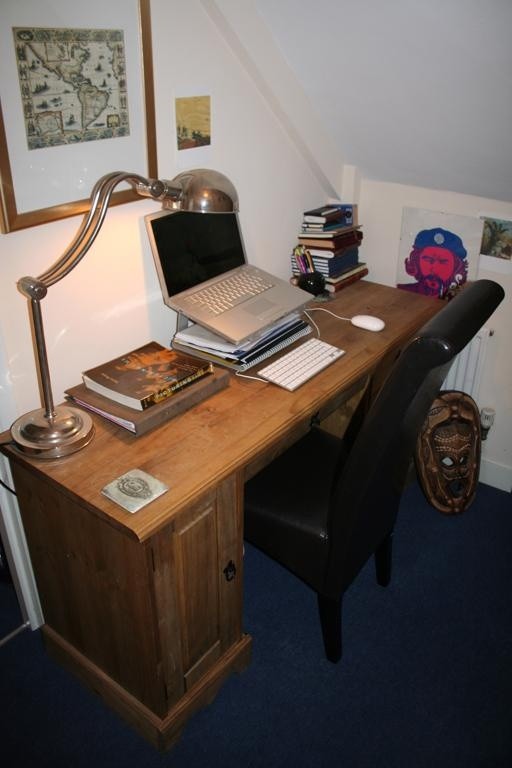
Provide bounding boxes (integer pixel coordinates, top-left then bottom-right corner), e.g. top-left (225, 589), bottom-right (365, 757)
top-left (1, 277), bottom-right (446, 756)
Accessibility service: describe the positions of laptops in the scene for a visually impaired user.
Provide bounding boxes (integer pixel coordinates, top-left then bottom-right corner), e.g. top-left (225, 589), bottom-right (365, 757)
top-left (145, 209), bottom-right (316, 345)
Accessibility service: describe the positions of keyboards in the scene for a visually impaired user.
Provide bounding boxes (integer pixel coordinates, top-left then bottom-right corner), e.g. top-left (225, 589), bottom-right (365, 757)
top-left (255, 338), bottom-right (349, 392)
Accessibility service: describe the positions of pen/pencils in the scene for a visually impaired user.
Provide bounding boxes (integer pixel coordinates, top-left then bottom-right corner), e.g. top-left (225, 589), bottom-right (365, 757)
top-left (293, 246), bottom-right (315, 275)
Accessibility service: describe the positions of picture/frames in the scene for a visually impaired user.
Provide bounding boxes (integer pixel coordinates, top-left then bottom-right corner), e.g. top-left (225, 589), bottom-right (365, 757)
top-left (0, 0), bottom-right (156, 233)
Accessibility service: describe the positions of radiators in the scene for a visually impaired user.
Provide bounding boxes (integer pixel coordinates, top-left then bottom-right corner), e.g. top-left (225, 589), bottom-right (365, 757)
top-left (440, 326), bottom-right (496, 441)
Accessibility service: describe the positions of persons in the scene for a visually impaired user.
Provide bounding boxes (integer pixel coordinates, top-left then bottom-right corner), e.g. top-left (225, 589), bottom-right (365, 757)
top-left (395, 225), bottom-right (469, 300)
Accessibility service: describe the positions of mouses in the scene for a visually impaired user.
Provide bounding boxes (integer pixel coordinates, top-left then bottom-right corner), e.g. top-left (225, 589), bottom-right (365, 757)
top-left (350, 314), bottom-right (386, 332)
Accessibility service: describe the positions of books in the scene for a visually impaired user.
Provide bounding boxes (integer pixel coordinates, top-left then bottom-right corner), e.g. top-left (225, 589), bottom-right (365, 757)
top-left (170, 304), bottom-right (315, 374)
top-left (290, 203), bottom-right (368, 295)
top-left (64, 368), bottom-right (232, 438)
top-left (81, 340), bottom-right (214, 410)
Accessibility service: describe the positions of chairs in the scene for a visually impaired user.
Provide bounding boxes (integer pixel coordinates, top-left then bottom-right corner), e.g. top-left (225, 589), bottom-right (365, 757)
top-left (247, 281), bottom-right (503, 663)
